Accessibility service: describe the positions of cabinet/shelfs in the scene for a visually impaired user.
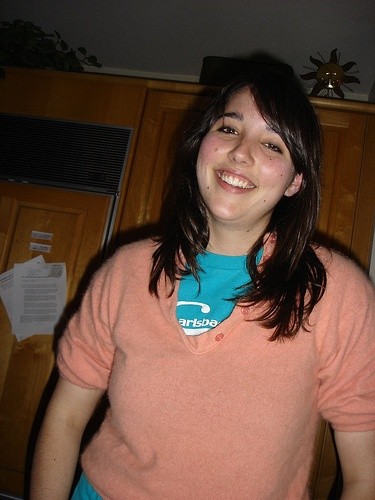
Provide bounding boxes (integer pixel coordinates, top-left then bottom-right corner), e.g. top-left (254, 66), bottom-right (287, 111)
top-left (0, 60), bottom-right (375, 500)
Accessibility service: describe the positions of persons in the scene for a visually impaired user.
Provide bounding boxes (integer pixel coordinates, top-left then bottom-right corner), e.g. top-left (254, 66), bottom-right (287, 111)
top-left (23, 53), bottom-right (373, 500)
